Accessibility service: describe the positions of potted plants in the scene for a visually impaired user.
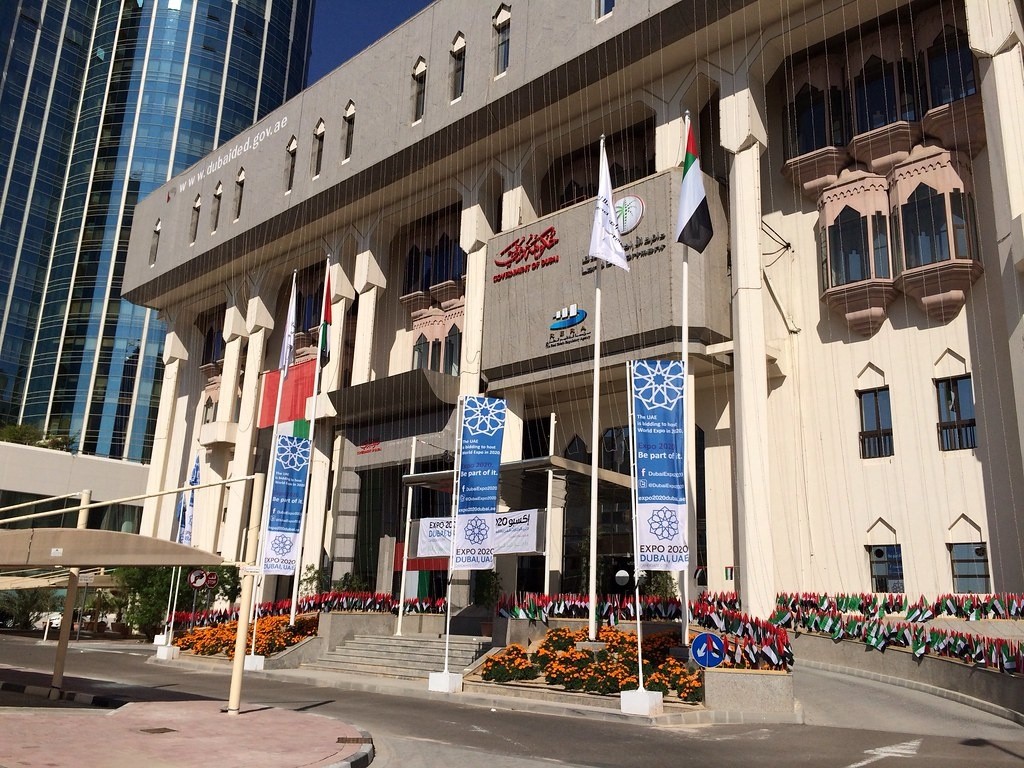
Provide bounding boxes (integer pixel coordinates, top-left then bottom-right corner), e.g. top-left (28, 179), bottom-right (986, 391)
top-left (111, 597), bottom-right (125, 631)
top-left (82, 594), bottom-right (97, 630)
top-left (97, 595), bottom-right (110, 632)
top-left (73, 590), bottom-right (84, 631)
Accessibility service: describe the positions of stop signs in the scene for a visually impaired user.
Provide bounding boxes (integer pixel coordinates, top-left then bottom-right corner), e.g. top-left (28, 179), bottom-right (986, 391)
top-left (206, 572), bottom-right (219, 589)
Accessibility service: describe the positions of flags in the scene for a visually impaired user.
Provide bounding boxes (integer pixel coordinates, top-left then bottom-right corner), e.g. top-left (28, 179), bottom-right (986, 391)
top-left (675, 123), bottom-right (713, 253)
top-left (689, 591), bottom-right (795, 670)
top-left (596, 594), bottom-right (636, 627)
top-left (639, 595), bottom-right (683, 623)
top-left (495, 591), bottom-right (590, 623)
top-left (296, 591), bottom-right (400, 617)
top-left (279, 284), bottom-right (295, 378)
top-left (254, 599), bottom-right (292, 618)
top-left (452, 397), bottom-right (506, 570)
top-left (632, 361), bottom-right (690, 571)
top-left (403, 597), bottom-right (448, 614)
top-left (770, 591), bottom-right (1024, 674)
top-left (261, 436), bottom-right (312, 575)
top-left (167, 608), bottom-right (239, 628)
top-left (589, 148), bottom-right (629, 272)
top-left (177, 456), bottom-right (200, 544)
top-left (317, 274), bottom-right (332, 367)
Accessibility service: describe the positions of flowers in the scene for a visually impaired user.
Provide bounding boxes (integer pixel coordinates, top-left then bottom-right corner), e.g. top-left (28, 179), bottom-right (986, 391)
top-left (481, 625), bottom-right (770, 702)
top-left (171, 614), bottom-right (318, 662)
